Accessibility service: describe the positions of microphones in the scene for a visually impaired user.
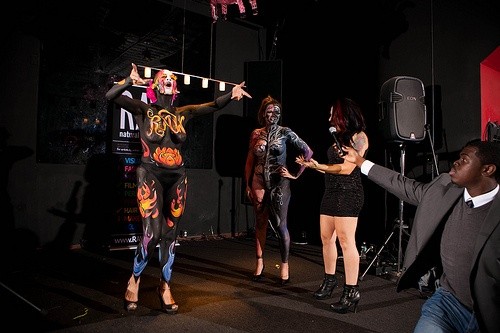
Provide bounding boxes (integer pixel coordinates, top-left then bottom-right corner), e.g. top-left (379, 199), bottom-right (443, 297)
top-left (328, 127), bottom-right (347, 157)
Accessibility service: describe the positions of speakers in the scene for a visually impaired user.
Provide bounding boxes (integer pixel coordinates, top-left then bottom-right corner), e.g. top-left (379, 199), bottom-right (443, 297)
top-left (378, 76), bottom-right (428, 146)
top-left (242, 59), bottom-right (283, 123)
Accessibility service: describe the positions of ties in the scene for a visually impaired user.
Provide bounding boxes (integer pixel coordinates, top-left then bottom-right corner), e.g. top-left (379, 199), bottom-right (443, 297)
top-left (466, 200), bottom-right (474, 208)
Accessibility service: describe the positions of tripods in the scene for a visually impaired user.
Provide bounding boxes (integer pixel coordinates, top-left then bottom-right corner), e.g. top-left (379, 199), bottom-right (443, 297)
top-left (360, 141), bottom-right (413, 280)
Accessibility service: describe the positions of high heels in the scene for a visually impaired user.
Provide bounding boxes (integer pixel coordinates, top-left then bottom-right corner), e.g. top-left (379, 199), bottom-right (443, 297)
top-left (329, 283), bottom-right (361, 313)
top-left (124, 281), bottom-right (138, 311)
top-left (280, 266), bottom-right (291, 285)
top-left (156, 286), bottom-right (178, 313)
top-left (252, 264), bottom-right (265, 281)
top-left (313, 273), bottom-right (336, 299)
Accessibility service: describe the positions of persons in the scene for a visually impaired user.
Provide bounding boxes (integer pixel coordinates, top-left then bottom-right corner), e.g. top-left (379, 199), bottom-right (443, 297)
top-left (105, 62), bottom-right (253, 316)
top-left (331, 139), bottom-right (500, 333)
top-left (245, 95), bottom-right (314, 288)
top-left (293, 104), bottom-right (370, 313)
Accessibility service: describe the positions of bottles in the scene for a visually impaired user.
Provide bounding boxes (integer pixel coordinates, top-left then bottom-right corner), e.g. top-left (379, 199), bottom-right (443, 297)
top-left (184, 230), bottom-right (188, 237)
top-left (361, 242), bottom-right (366, 260)
